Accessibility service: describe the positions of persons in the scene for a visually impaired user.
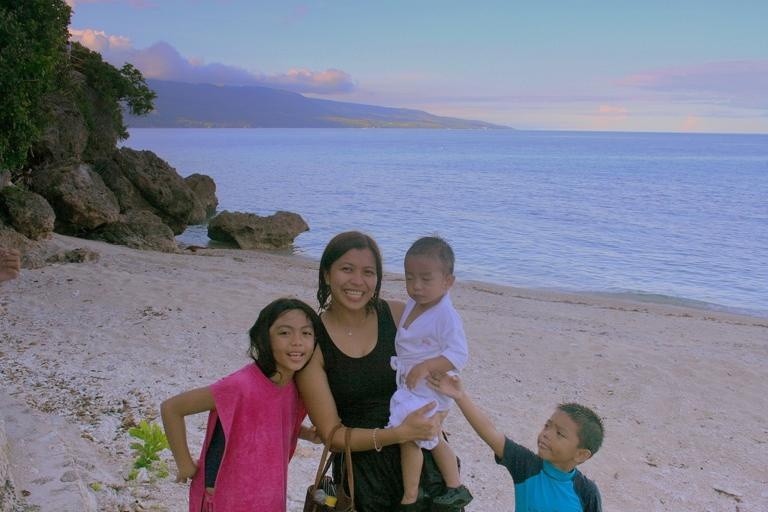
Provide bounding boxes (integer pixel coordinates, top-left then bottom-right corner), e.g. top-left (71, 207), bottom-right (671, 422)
top-left (424, 363), bottom-right (604, 512)
top-left (159, 297), bottom-right (324, 510)
top-left (294, 229), bottom-right (467, 511)
top-left (382, 233), bottom-right (476, 512)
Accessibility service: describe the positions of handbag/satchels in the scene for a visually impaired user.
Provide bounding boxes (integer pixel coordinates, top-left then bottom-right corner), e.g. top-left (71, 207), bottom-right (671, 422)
top-left (303, 423), bottom-right (356, 512)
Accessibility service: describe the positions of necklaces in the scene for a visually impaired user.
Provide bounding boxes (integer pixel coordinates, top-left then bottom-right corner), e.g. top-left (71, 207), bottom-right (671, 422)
top-left (346, 329), bottom-right (355, 337)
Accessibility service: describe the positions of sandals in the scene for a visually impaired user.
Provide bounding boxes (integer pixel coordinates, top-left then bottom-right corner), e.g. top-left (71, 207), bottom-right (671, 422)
top-left (394, 484), bottom-right (474, 512)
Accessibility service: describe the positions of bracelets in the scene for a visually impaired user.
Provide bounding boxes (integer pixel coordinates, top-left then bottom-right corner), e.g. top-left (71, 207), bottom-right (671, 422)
top-left (372, 426), bottom-right (383, 453)
